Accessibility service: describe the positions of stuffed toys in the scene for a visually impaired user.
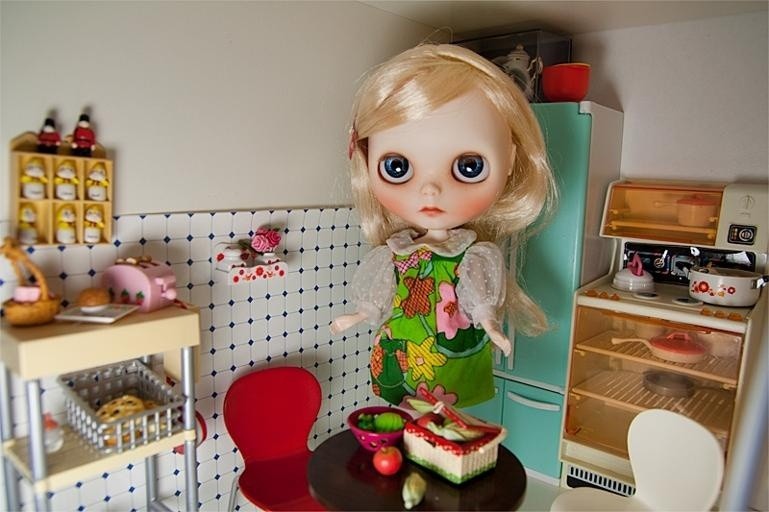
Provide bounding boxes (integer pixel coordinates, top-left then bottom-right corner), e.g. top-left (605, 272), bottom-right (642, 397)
top-left (16, 112), bottom-right (110, 246)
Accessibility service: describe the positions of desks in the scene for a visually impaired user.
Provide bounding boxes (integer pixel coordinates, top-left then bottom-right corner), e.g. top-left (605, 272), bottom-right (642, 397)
top-left (306, 427), bottom-right (527, 511)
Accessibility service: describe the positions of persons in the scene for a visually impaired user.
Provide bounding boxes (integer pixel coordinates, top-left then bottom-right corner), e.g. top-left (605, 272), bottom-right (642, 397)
top-left (327, 42), bottom-right (564, 412)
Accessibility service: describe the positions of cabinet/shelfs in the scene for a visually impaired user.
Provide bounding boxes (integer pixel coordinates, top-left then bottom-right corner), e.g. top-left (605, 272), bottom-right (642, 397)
top-left (559, 176), bottom-right (769, 499)
top-left (0, 299), bottom-right (202, 511)
top-left (447, 102), bottom-right (625, 486)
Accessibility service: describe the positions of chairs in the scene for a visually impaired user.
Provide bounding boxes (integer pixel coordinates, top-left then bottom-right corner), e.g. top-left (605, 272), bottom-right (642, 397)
top-left (222, 367), bottom-right (324, 512)
top-left (546, 407), bottom-right (723, 510)
